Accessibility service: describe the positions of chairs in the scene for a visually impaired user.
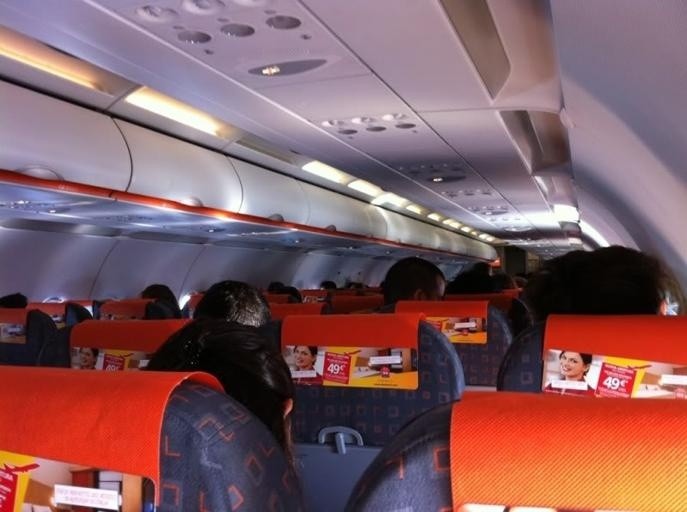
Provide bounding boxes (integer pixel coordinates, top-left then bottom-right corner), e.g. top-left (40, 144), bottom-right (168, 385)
top-left (0, 291), bottom-right (687, 512)
